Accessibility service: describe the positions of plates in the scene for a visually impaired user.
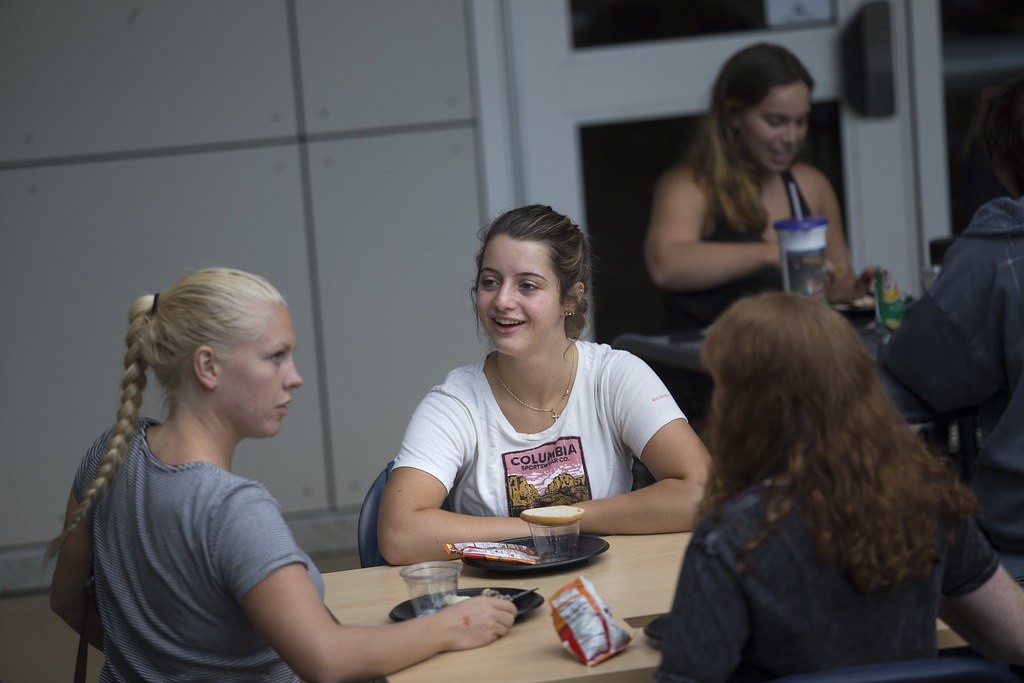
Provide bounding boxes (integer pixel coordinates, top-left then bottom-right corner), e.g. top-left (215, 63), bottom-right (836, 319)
top-left (465, 536), bottom-right (608, 576)
top-left (389, 586), bottom-right (545, 626)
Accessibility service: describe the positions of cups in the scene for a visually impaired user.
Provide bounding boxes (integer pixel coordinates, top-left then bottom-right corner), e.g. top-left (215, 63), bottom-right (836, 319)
top-left (526, 517), bottom-right (580, 561)
top-left (773, 219), bottom-right (830, 304)
top-left (398, 561), bottom-right (466, 617)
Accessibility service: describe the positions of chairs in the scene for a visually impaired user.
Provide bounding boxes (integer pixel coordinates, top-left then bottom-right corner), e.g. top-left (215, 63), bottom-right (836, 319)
top-left (358, 461), bottom-right (389, 567)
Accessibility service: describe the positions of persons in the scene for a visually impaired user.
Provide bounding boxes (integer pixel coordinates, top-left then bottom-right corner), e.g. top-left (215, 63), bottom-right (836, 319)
top-left (48, 267), bottom-right (517, 683)
top-left (879, 73), bottom-right (1023, 551)
top-left (644, 44), bottom-right (895, 399)
top-left (379, 205), bottom-right (713, 567)
top-left (658, 293), bottom-right (1024, 683)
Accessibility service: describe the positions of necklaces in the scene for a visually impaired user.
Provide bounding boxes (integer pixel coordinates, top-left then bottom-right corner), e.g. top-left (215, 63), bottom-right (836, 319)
top-left (494, 341), bottom-right (575, 422)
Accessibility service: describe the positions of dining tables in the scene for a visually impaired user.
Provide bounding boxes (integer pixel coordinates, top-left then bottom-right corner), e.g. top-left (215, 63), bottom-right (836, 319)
top-left (315, 530), bottom-right (970, 683)
top-left (613, 294), bottom-right (918, 418)
